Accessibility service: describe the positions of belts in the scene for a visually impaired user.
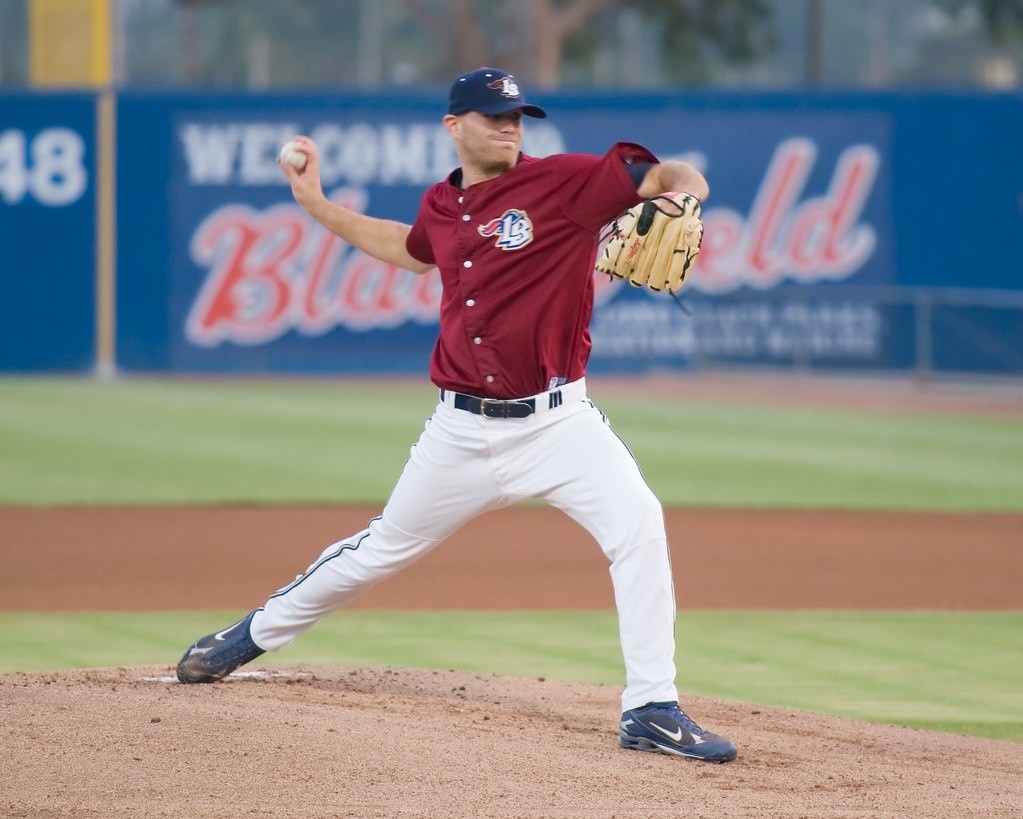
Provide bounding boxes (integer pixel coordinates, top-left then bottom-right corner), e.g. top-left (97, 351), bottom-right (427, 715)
top-left (441, 389), bottom-right (562, 419)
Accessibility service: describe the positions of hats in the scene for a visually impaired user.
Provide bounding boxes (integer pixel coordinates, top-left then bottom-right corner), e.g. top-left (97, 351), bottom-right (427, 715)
top-left (448, 67), bottom-right (547, 120)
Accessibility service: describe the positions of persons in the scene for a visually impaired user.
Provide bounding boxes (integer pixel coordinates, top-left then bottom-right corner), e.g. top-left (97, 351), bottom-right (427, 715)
top-left (177, 66), bottom-right (737, 765)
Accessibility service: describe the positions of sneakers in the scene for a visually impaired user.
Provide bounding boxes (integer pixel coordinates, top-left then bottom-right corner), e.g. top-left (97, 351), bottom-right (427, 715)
top-left (620, 702), bottom-right (737, 762)
top-left (176, 611), bottom-right (267, 684)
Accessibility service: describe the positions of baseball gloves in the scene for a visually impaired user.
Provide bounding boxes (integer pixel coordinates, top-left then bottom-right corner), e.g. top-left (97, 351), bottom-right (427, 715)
top-left (595, 190), bottom-right (704, 293)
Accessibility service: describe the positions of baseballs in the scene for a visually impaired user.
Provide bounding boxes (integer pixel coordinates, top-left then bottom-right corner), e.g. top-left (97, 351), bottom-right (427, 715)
top-left (280, 140), bottom-right (307, 170)
